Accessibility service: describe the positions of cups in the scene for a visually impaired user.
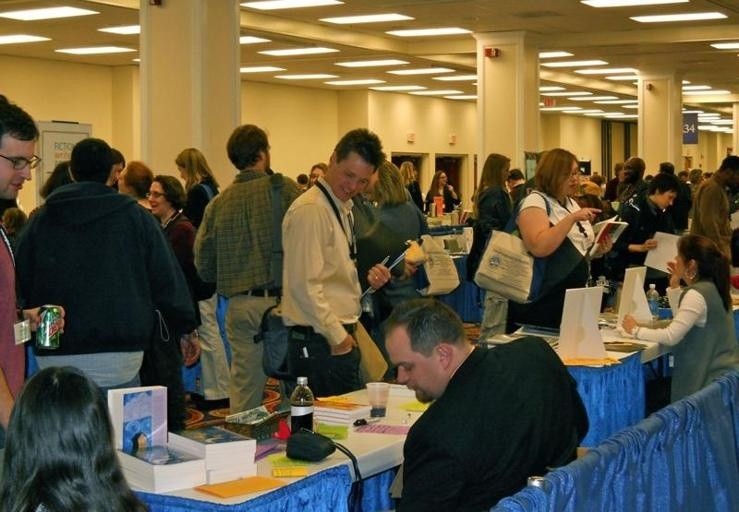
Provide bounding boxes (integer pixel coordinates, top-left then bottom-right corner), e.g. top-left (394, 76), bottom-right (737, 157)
top-left (366, 382), bottom-right (390, 417)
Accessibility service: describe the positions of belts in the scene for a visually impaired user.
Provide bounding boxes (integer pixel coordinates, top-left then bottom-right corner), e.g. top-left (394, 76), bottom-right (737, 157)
top-left (243, 288), bottom-right (283, 296)
top-left (292, 323), bottom-right (358, 334)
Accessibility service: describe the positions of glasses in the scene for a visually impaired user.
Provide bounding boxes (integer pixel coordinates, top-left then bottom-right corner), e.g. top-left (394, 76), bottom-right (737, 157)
top-left (146, 192), bottom-right (167, 198)
top-left (0, 155), bottom-right (41, 170)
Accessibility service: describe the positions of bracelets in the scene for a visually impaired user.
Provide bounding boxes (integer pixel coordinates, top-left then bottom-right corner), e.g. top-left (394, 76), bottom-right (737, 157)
top-left (190, 330), bottom-right (199, 338)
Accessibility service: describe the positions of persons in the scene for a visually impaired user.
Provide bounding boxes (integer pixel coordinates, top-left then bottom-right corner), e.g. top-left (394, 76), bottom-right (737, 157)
top-left (619, 234), bottom-right (739, 403)
top-left (1, 94), bottom-right (66, 440)
top-left (384, 295), bottom-right (590, 510)
top-left (277, 127), bottom-right (390, 396)
top-left (0, 362), bottom-right (153, 511)
top-left (0, 123), bottom-right (739, 413)
top-left (13, 134), bottom-right (202, 403)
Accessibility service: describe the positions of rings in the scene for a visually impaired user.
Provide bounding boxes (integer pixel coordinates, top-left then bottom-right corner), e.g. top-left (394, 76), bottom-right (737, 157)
top-left (373, 274), bottom-right (379, 281)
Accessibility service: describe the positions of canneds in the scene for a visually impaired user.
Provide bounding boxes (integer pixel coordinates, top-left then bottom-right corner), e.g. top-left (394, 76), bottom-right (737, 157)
top-left (35, 305), bottom-right (61, 349)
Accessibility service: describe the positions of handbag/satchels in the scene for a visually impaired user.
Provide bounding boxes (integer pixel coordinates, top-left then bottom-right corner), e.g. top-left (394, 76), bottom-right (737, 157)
top-left (416, 234), bottom-right (460, 296)
top-left (254, 306), bottom-right (291, 382)
top-left (474, 230), bottom-right (535, 304)
top-left (154, 312), bottom-right (184, 369)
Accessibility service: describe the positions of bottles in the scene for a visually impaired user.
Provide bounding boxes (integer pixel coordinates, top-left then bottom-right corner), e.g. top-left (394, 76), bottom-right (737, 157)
top-left (290, 376), bottom-right (314, 435)
top-left (430, 203), bottom-right (437, 218)
top-left (646, 284), bottom-right (660, 320)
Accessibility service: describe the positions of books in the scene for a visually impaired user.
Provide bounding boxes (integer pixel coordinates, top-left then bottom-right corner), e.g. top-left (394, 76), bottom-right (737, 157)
top-left (309, 396), bottom-right (374, 428)
top-left (109, 383), bottom-right (262, 492)
top-left (485, 322), bottom-right (564, 352)
